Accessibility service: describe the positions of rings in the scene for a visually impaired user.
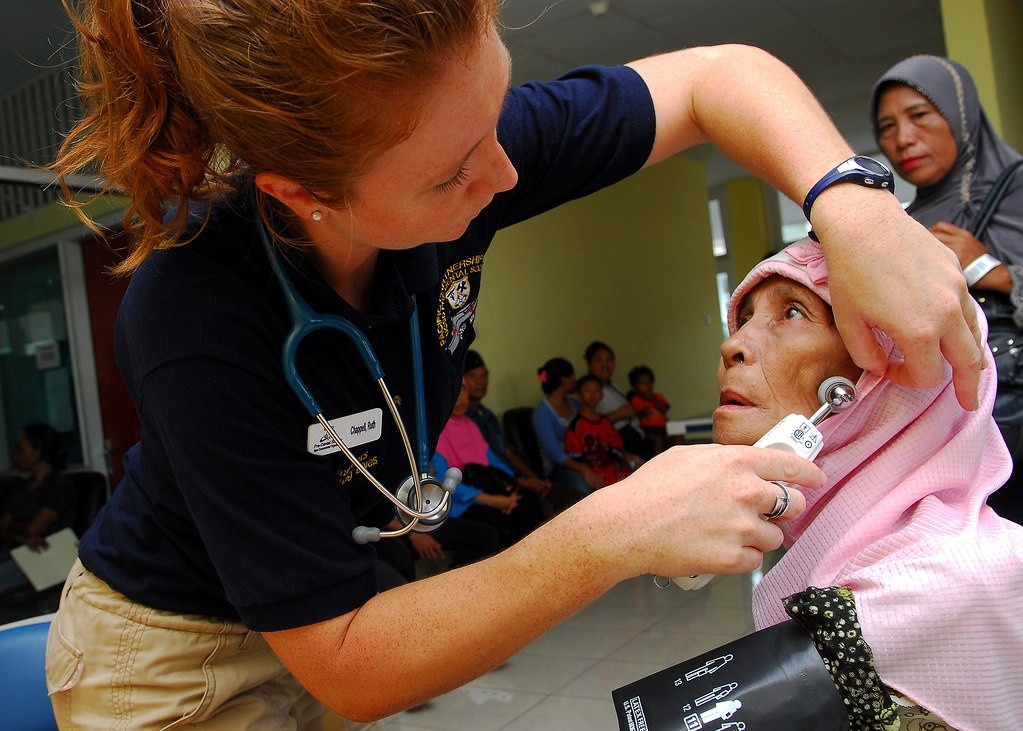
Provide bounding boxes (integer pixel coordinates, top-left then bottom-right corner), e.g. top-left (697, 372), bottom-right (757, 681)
top-left (762, 482), bottom-right (791, 518)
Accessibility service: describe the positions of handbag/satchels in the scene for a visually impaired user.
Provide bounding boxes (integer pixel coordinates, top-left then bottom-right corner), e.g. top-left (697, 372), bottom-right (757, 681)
top-left (946, 159), bottom-right (1023, 455)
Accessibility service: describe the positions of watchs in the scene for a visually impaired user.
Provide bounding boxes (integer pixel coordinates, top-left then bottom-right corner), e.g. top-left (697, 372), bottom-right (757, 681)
top-left (804, 155), bottom-right (895, 244)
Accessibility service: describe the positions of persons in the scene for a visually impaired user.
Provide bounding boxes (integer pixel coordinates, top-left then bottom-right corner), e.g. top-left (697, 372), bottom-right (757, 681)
top-left (0, 423), bottom-right (72, 597)
top-left (378, 514), bottom-right (443, 590)
top-left (432, 376), bottom-right (522, 519)
top-left (46, 0), bottom-right (990, 731)
top-left (630, 366), bottom-right (670, 434)
top-left (564, 374), bottom-right (631, 484)
top-left (585, 341), bottom-right (644, 437)
top-left (868, 54), bottom-right (1023, 525)
top-left (461, 349), bottom-right (547, 493)
top-left (713, 237), bottom-right (1023, 731)
top-left (533, 357), bottom-right (646, 494)
